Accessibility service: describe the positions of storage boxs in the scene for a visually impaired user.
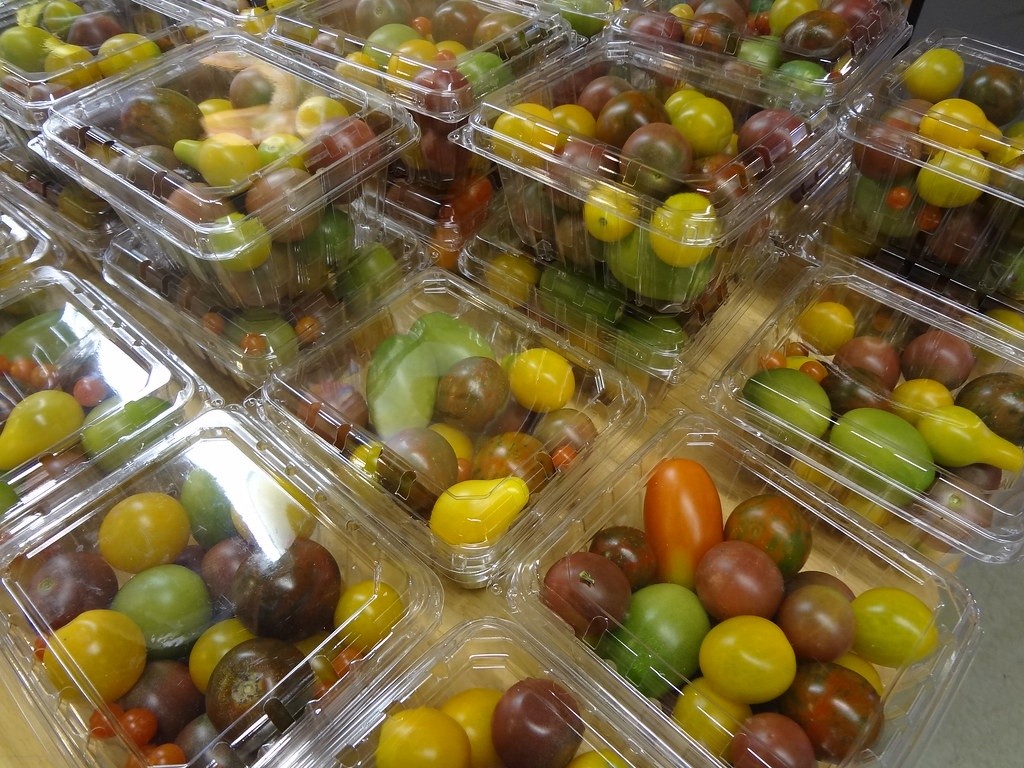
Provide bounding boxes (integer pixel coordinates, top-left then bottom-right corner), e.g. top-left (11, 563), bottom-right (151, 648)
top-left (1, 1), bottom-right (1024, 768)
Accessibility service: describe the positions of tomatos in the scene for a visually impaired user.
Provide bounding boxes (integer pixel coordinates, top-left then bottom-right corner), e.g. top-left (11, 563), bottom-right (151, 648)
top-left (0, 0), bottom-right (1023, 768)
